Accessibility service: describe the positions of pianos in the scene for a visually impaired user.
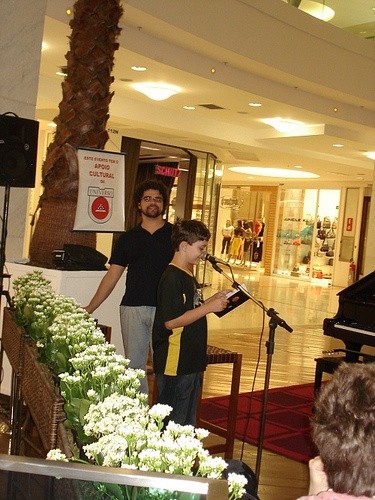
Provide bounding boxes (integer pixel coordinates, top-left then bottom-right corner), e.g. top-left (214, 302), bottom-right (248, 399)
top-left (322, 270), bottom-right (375, 359)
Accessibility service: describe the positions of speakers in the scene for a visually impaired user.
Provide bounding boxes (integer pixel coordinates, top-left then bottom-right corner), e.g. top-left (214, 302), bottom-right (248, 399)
top-left (1, 114), bottom-right (39, 187)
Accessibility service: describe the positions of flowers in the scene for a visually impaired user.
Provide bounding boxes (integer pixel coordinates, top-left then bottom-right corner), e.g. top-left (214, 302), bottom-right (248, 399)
top-left (12, 270), bottom-right (248, 500)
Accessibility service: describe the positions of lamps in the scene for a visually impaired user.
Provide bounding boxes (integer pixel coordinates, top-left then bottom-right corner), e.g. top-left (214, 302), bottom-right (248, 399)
top-left (131, 82), bottom-right (180, 100)
top-left (284, 0), bottom-right (336, 23)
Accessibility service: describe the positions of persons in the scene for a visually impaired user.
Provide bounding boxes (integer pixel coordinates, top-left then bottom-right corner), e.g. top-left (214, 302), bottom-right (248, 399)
top-left (220, 218), bottom-right (234, 261)
top-left (226, 227), bottom-right (242, 265)
top-left (294, 362), bottom-right (375, 500)
top-left (83, 179), bottom-right (176, 398)
top-left (237, 228), bottom-right (252, 267)
top-left (152, 216), bottom-right (229, 431)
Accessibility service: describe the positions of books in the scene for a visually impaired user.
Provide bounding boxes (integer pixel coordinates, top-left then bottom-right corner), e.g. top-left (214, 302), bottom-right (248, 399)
top-left (212, 283), bottom-right (250, 319)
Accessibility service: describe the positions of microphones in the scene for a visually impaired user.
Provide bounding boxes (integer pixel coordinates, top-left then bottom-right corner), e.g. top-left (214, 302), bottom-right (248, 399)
top-left (201, 251), bottom-right (228, 266)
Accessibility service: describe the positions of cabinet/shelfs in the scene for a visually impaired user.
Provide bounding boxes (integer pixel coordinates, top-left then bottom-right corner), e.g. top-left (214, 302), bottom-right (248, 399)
top-left (288, 220), bottom-right (336, 277)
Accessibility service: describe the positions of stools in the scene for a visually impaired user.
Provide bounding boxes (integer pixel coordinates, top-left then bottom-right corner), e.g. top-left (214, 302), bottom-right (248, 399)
top-left (194, 343), bottom-right (243, 467)
top-left (313, 354), bottom-right (364, 408)
